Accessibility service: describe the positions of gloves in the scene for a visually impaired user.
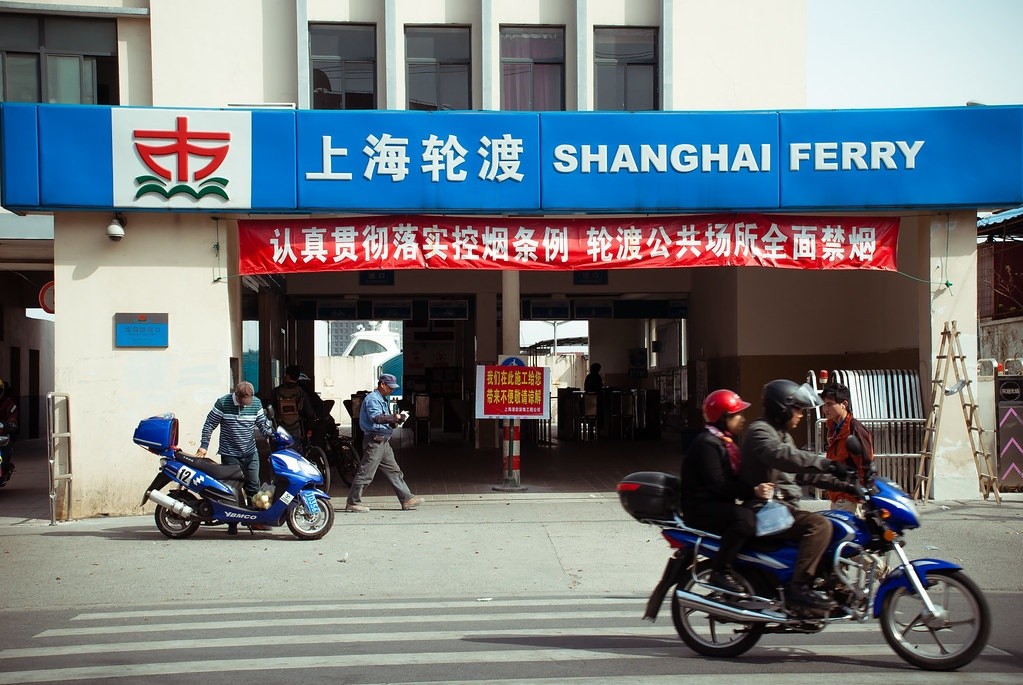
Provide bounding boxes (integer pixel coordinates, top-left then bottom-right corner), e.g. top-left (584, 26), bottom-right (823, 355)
top-left (829, 460), bottom-right (855, 481)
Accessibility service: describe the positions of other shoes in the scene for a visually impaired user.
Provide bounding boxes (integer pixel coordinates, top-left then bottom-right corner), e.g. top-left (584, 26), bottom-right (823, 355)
top-left (228, 524), bottom-right (237, 535)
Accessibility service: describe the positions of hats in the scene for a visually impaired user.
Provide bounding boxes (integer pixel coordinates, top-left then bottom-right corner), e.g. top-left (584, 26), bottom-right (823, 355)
top-left (380, 374), bottom-right (400, 388)
top-left (236, 381), bottom-right (254, 404)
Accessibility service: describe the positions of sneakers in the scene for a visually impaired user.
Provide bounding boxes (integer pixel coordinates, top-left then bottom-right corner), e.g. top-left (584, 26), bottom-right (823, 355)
top-left (402, 498), bottom-right (425, 509)
top-left (346, 504), bottom-right (371, 513)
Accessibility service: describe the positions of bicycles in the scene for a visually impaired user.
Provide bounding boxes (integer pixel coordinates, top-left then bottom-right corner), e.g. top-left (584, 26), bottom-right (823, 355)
top-left (299, 417), bottom-right (362, 495)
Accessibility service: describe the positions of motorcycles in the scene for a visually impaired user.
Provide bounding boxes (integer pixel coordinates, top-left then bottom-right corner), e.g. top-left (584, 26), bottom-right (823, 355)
top-left (617, 435), bottom-right (992, 672)
top-left (0, 405), bottom-right (16, 487)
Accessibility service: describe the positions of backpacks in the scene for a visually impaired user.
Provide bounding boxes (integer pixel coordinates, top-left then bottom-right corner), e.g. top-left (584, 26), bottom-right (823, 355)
top-left (277, 384), bottom-right (299, 429)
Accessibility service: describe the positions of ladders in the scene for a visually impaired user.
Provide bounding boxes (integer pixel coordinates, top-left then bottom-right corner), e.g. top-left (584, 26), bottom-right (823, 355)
top-left (911, 320), bottom-right (1003, 507)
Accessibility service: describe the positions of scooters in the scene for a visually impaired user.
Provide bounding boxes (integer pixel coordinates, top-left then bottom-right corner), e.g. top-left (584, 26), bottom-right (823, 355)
top-left (133, 405), bottom-right (334, 539)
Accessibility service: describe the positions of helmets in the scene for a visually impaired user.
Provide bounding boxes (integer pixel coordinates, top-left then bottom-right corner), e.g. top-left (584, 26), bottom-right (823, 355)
top-left (763, 380), bottom-right (817, 424)
top-left (703, 389), bottom-right (752, 423)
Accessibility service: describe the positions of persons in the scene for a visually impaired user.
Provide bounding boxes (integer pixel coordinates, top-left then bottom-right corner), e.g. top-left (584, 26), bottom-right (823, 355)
top-left (584, 363), bottom-right (605, 439)
top-left (822, 383), bottom-right (874, 588)
top-left (273, 365), bottom-right (310, 418)
top-left (0, 379), bottom-right (18, 482)
top-left (345, 374), bottom-right (425, 513)
top-left (737, 380), bottom-right (864, 608)
top-left (680, 390), bottom-right (775, 593)
top-left (195, 381), bottom-right (276, 535)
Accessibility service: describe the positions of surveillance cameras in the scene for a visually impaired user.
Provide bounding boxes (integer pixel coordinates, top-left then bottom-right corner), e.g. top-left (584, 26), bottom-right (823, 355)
top-left (105, 220), bottom-right (125, 242)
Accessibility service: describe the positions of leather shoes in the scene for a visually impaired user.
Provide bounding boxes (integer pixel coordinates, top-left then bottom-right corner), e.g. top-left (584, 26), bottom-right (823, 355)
top-left (788, 583), bottom-right (838, 608)
top-left (708, 572), bottom-right (744, 591)
